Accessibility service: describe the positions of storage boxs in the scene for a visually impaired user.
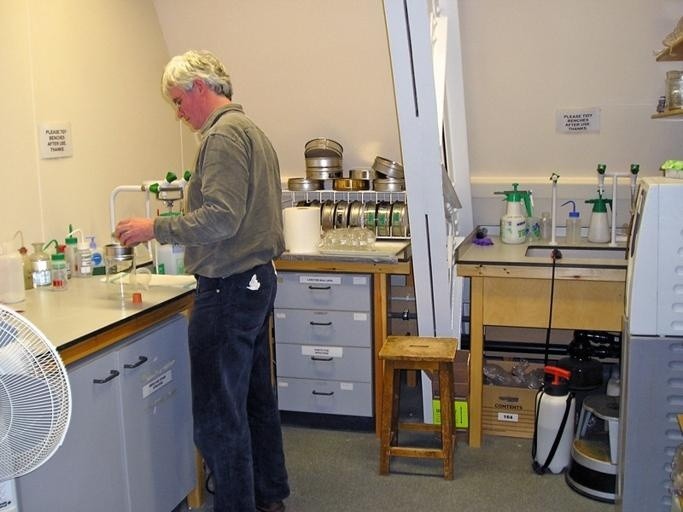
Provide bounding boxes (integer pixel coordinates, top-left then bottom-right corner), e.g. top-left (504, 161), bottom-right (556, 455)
top-left (481, 358), bottom-right (547, 441)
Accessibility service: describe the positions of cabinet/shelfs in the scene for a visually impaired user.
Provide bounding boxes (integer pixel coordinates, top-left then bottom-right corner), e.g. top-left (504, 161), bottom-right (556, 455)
top-left (273, 272), bottom-right (375, 418)
top-left (14, 312), bottom-right (198, 512)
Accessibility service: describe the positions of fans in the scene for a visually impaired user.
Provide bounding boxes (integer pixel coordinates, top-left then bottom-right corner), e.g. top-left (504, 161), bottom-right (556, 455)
top-left (0, 304), bottom-right (73, 512)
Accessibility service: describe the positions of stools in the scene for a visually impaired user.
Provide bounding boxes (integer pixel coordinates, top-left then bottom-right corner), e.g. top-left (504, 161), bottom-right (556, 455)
top-left (377, 335), bottom-right (459, 482)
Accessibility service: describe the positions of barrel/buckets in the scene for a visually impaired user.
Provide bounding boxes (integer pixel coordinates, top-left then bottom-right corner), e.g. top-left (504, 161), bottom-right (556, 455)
top-left (532, 365), bottom-right (577, 475)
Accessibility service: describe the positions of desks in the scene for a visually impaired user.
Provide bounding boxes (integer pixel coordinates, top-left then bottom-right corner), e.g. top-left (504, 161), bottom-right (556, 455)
top-left (456, 264), bottom-right (626, 450)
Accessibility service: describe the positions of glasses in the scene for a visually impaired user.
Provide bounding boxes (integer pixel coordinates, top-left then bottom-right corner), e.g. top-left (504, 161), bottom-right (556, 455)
top-left (169, 84), bottom-right (196, 113)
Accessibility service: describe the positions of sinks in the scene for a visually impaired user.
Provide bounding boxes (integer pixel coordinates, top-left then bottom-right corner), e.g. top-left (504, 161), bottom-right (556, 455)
top-left (525, 245), bottom-right (627, 259)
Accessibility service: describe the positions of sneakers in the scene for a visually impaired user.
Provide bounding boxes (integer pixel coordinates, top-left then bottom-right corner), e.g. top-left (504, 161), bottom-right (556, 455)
top-left (257, 500), bottom-right (287, 512)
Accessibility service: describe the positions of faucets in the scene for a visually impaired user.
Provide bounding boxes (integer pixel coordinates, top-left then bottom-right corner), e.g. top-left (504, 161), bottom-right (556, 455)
top-left (597, 163), bottom-right (606, 196)
top-left (631, 164), bottom-right (639, 194)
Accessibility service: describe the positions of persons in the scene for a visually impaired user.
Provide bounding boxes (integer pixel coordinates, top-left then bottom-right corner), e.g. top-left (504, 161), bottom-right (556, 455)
top-left (112, 46), bottom-right (290, 511)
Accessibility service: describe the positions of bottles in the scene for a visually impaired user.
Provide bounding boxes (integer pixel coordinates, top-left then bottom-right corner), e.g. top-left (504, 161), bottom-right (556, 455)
top-left (560, 200), bottom-right (581, 244)
top-left (540, 211), bottom-right (553, 241)
top-left (1, 223), bottom-right (136, 303)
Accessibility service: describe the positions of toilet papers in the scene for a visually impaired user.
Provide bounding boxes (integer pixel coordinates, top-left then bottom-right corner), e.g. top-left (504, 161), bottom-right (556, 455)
top-left (282, 207), bottom-right (321, 256)
